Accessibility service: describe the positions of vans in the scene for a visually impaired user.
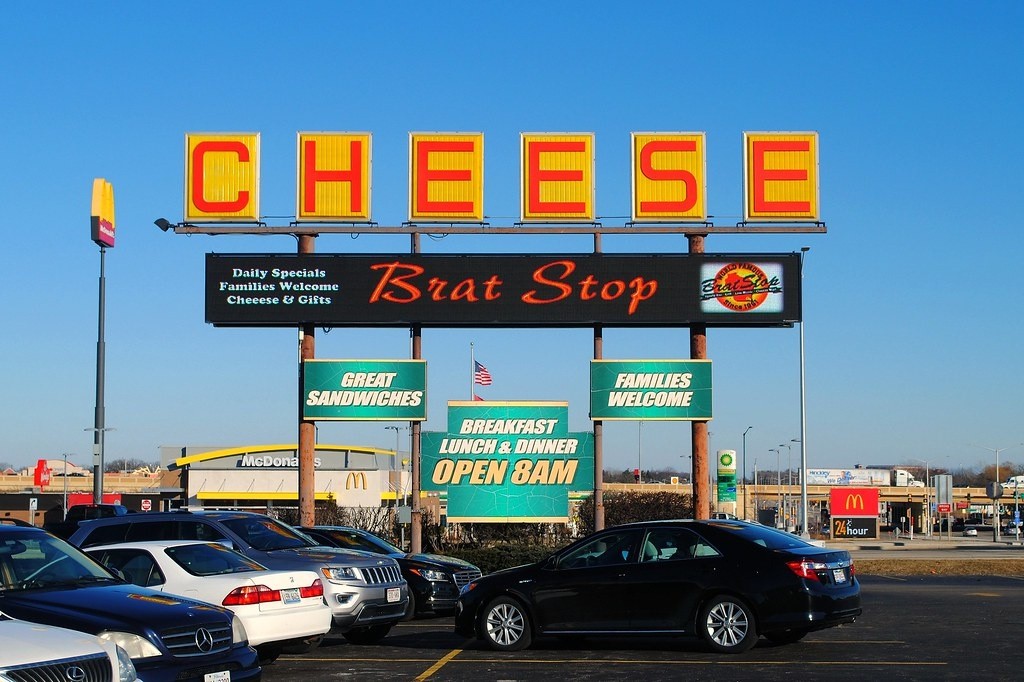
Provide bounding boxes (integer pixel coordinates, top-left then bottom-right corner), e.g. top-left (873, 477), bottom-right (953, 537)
top-left (712, 512), bottom-right (738, 520)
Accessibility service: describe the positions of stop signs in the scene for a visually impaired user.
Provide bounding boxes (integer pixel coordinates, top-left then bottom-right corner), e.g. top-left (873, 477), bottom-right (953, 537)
top-left (142, 500), bottom-right (151, 510)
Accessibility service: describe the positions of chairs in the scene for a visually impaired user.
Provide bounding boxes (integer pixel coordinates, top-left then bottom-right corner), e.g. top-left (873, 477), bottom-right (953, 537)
top-left (668, 540), bottom-right (692, 559)
top-left (646, 540), bottom-right (662, 561)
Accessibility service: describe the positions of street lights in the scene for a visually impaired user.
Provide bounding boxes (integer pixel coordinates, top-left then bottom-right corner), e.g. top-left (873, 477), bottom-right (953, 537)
top-left (385, 425), bottom-right (406, 507)
top-left (969, 443), bottom-right (1023, 536)
top-left (769, 448), bottom-right (780, 527)
top-left (743, 426), bottom-right (754, 520)
top-left (907, 455), bottom-right (950, 537)
top-left (780, 443), bottom-right (791, 526)
top-left (708, 431), bottom-right (713, 504)
top-left (800, 246), bottom-right (809, 538)
top-left (63, 452), bottom-right (75, 519)
top-left (679, 455), bottom-right (693, 484)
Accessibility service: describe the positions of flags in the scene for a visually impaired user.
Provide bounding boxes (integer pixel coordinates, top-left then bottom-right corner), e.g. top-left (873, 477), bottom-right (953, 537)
top-left (473, 360), bottom-right (491, 385)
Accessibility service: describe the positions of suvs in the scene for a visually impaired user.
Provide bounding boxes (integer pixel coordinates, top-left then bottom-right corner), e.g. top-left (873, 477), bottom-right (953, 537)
top-left (0, 517), bottom-right (261, 682)
top-left (49, 510), bottom-right (410, 653)
top-left (1, 612), bottom-right (144, 682)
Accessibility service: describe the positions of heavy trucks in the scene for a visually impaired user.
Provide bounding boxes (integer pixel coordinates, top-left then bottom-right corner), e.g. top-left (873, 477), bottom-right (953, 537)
top-left (1001, 475), bottom-right (1024, 489)
top-left (798, 468), bottom-right (924, 487)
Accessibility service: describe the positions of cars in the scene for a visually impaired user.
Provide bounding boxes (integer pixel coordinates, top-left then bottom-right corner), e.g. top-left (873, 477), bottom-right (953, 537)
top-left (453, 519), bottom-right (863, 653)
top-left (19, 540), bottom-right (331, 666)
top-left (821, 526), bottom-right (830, 534)
top-left (249, 525), bottom-right (482, 621)
top-left (963, 527), bottom-right (978, 537)
top-left (1004, 525), bottom-right (1021, 535)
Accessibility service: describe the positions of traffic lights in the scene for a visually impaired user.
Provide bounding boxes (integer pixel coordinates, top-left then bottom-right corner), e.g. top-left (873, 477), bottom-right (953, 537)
top-left (1000, 506), bottom-right (1003, 513)
top-left (1014, 491), bottom-right (1016, 499)
